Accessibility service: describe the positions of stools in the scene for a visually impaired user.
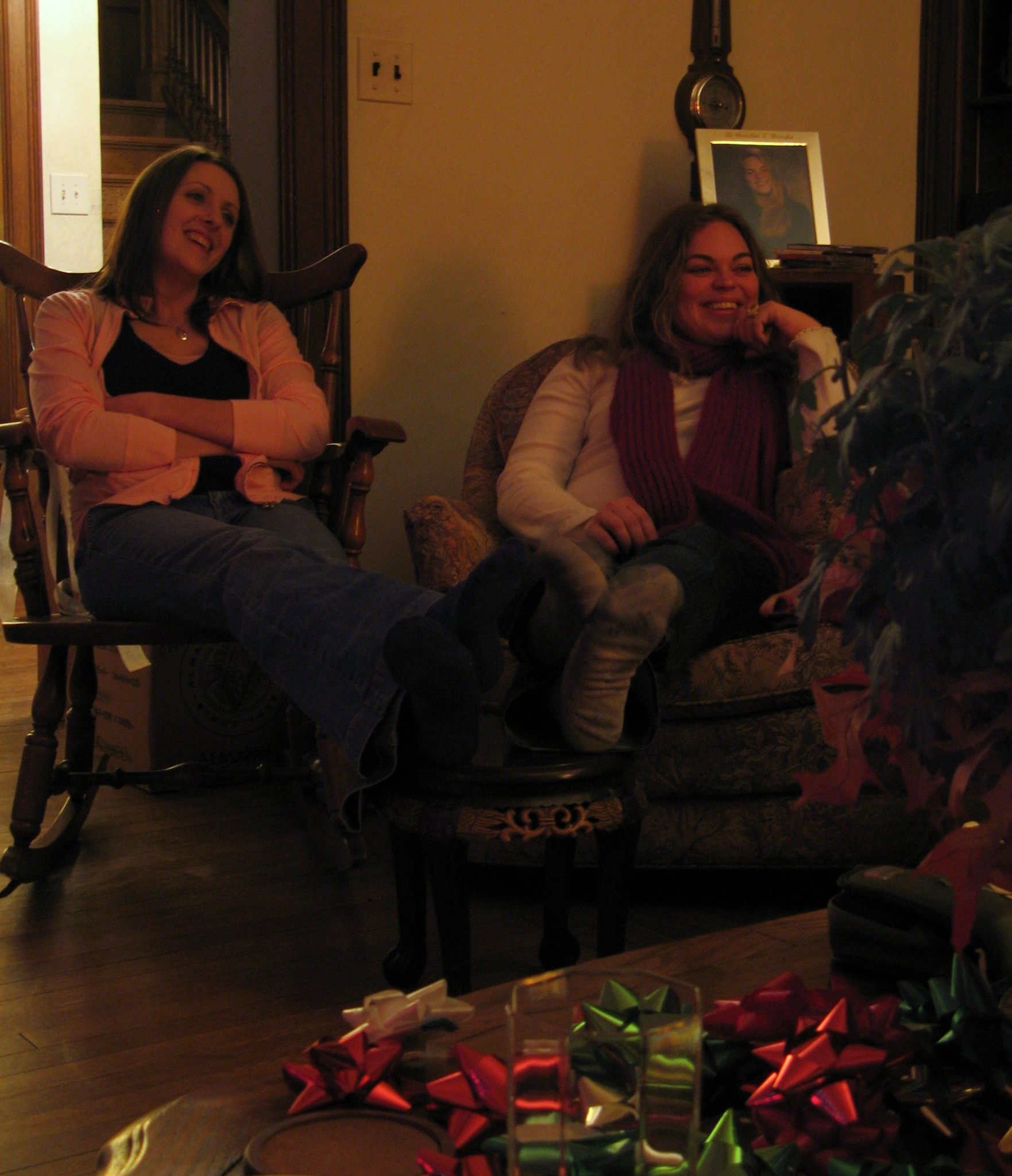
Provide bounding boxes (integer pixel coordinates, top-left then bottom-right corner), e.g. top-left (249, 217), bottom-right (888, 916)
top-left (383, 709), bottom-right (643, 994)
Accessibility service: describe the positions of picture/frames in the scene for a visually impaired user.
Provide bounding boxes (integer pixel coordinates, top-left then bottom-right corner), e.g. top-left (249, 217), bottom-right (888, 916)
top-left (695, 128), bottom-right (830, 267)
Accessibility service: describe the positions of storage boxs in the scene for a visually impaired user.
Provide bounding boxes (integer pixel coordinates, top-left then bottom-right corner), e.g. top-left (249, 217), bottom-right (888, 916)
top-left (37, 644), bottom-right (289, 792)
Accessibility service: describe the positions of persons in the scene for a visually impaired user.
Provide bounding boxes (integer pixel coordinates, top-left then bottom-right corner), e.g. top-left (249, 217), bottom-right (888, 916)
top-left (735, 147), bottom-right (817, 255)
top-left (27, 147), bottom-right (544, 781)
top-left (496, 200), bottom-right (856, 753)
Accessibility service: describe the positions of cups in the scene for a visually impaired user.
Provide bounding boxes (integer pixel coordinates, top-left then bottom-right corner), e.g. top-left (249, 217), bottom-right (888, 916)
top-left (507, 966), bottom-right (704, 1176)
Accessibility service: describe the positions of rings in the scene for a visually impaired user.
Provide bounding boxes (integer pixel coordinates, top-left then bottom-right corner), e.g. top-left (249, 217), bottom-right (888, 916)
top-left (746, 303), bottom-right (760, 318)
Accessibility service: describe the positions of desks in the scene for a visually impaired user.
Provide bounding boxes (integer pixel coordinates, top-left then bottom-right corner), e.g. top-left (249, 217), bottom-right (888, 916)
top-left (96, 908), bottom-right (832, 1176)
top-left (768, 269), bottom-right (904, 329)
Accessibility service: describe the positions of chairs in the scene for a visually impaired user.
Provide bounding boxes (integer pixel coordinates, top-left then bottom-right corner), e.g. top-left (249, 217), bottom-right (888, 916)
top-left (0, 240), bottom-right (407, 899)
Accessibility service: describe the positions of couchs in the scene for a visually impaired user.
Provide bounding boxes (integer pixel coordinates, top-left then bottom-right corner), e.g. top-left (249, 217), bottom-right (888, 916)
top-left (402, 336), bottom-right (930, 868)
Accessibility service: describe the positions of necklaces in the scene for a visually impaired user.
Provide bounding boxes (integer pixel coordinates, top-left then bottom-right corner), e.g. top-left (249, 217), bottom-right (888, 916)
top-left (152, 317), bottom-right (192, 342)
